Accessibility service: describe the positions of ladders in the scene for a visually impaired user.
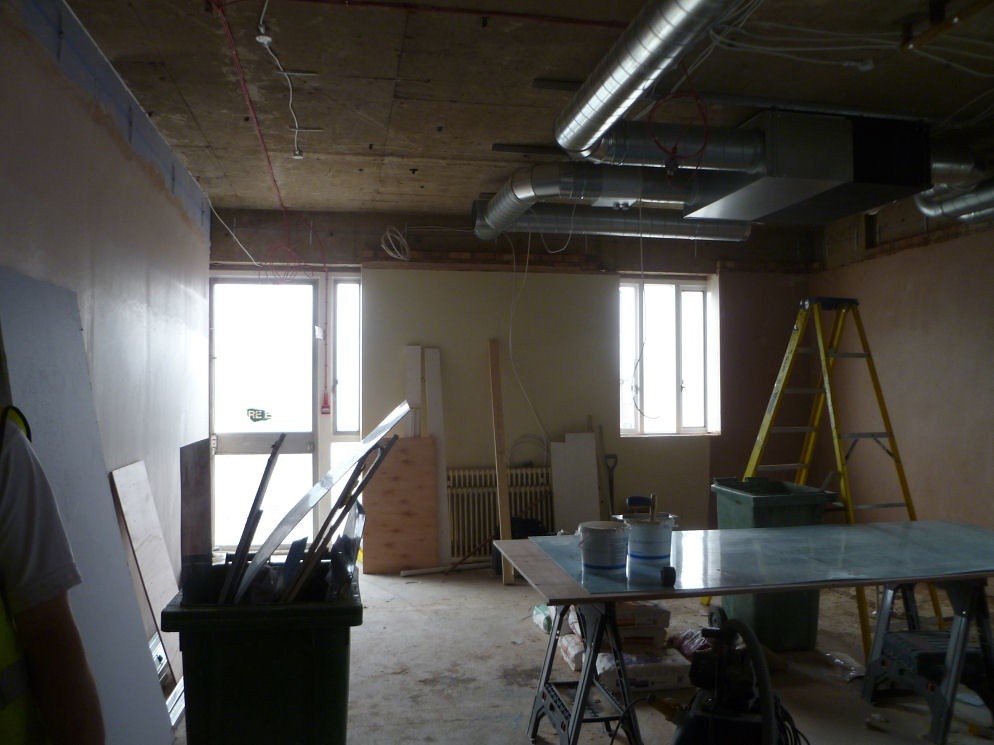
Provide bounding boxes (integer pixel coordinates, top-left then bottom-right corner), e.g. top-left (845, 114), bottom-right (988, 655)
top-left (750, 291), bottom-right (946, 678)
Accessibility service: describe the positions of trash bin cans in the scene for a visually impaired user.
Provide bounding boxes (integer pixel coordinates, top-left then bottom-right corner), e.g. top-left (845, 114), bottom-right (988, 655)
top-left (706, 471), bottom-right (850, 653)
top-left (155, 550), bottom-right (369, 745)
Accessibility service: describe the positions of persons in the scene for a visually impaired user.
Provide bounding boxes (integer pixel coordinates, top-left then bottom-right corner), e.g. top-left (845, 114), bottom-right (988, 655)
top-left (0, 402), bottom-right (106, 745)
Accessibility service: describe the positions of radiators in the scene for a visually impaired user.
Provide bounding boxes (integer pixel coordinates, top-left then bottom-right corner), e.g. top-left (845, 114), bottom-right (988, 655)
top-left (447, 469), bottom-right (555, 562)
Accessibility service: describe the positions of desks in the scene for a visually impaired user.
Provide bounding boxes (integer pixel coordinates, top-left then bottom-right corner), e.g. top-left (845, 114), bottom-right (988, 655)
top-left (491, 536), bottom-right (994, 745)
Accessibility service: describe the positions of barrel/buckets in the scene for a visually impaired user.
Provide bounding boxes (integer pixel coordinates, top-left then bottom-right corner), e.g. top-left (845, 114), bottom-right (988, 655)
top-left (626, 513), bottom-right (672, 567)
top-left (577, 520), bottom-right (629, 575)
